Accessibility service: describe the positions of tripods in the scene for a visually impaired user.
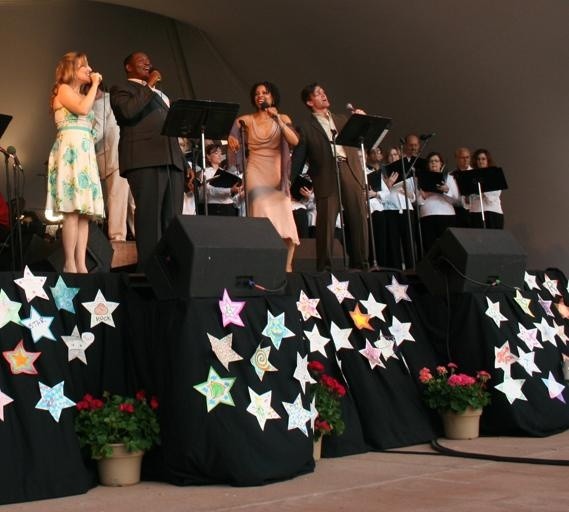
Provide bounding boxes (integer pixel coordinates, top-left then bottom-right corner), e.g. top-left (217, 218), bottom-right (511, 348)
top-left (360, 137), bottom-right (403, 273)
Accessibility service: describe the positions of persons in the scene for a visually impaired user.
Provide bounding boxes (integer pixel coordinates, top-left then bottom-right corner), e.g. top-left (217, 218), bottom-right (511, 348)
top-left (173, 135), bottom-right (248, 214)
top-left (227, 80), bottom-right (301, 272)
top-left (82, 79), bottom-right (137, 242)
top-left (46, 50), bottom-right (107, 274)
top-left (109, 49), bottom-right (197, 263)
top-left (291, 82), bottom-right (370, 271)
top-left (292, 134), bottom-right (504, 265)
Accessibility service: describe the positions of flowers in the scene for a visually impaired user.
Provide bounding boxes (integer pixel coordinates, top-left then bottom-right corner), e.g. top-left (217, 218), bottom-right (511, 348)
top-left (72, 390), bottom-right (161, 459)
top-left (308, 361), bottom-right (346, 440)
top-left (418, 363), bottom-right (492, 417)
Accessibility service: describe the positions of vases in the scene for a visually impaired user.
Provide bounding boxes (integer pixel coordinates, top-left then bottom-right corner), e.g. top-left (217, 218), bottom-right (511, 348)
top-left (313, 437), bottom-right (322, 462)
top-left (94, 443), bottom-right (144, 486)
top-left (440, 407), bottom-right (483, 440)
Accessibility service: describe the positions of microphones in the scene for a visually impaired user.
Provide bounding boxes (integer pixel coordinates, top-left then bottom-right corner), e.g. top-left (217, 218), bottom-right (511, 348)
top-left (345, 103), bottom-right (355, 112)
top-left (261, 102), bottom-right (277, 119)
top-left (148, 67), bottom-right (162, 83)
top-left (420, 133), bottom-right (436, 140)
top-left (7, 146), bottom-right (23, 172)
